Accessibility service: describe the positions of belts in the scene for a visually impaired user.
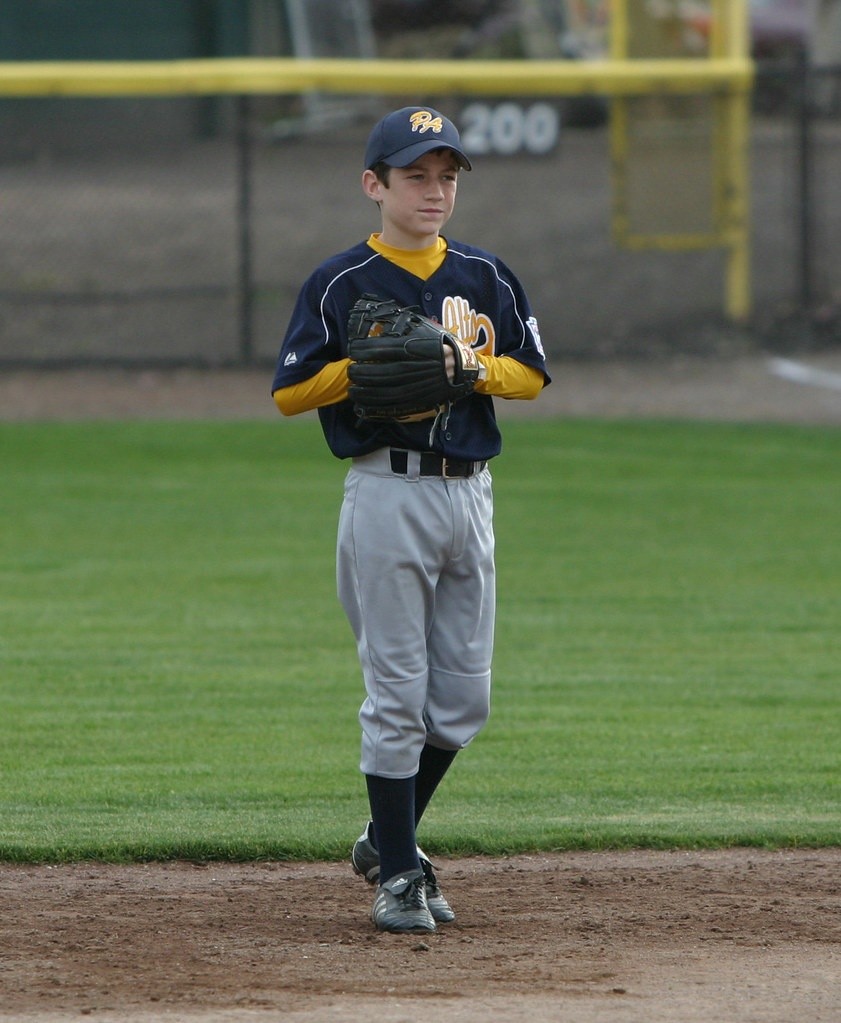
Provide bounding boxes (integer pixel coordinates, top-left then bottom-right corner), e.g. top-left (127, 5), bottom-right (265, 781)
top-left (390, 451), bottom-right (485, 477)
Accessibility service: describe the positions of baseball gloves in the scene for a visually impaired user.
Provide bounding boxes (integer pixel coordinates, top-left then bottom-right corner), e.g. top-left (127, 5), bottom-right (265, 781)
top-left (345, 292), bottom-right (479, 422)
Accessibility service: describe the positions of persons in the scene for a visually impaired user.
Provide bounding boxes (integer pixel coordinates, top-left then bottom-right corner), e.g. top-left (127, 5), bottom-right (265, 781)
top-left (268, 106), bottom-right (551, 933)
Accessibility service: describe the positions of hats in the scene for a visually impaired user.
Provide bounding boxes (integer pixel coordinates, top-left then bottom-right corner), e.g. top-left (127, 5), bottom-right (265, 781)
top-left (364, 107), bottom-right (471, 172)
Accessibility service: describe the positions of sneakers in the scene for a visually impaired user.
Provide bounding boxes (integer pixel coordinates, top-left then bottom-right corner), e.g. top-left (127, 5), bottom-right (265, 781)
top-left (373, 870), bottom-right (438, 933)
top-left (351, 821), bottom-right (456, 922)
